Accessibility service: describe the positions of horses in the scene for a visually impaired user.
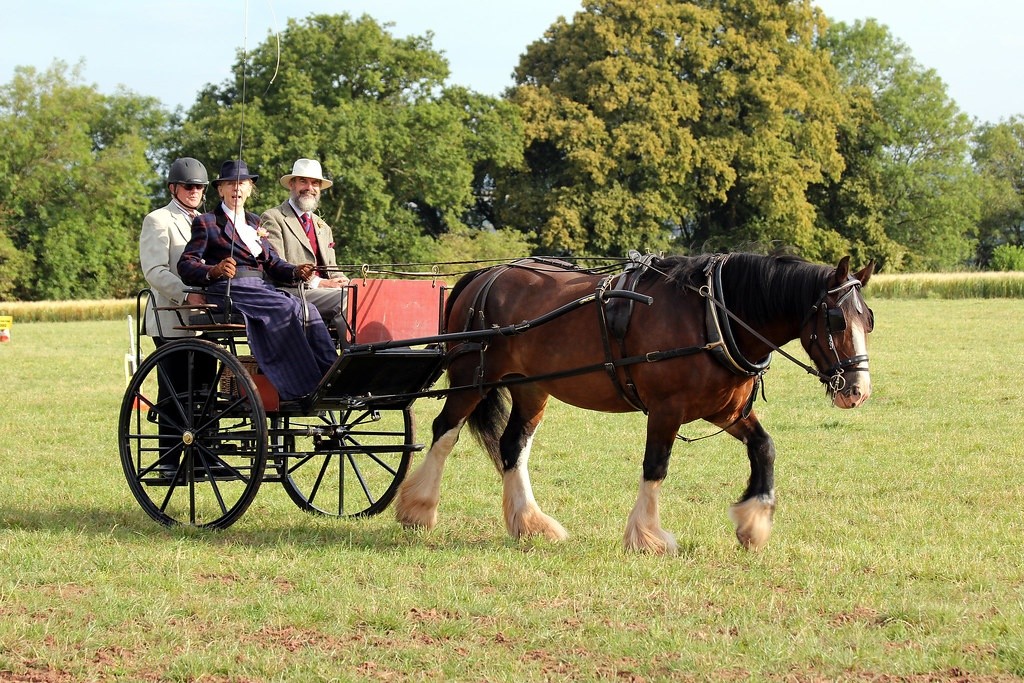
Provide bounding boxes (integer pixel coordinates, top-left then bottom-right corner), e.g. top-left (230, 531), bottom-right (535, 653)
top-left (391, 240), bottom-right (875, 556)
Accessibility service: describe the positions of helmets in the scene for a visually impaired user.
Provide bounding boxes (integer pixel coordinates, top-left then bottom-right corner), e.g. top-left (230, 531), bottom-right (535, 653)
top-left (166, 157), bottom-right (210, 185)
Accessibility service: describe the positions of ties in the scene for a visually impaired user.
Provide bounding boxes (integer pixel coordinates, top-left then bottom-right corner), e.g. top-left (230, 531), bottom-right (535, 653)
top-left (302, 214), bottom-right (311, 233)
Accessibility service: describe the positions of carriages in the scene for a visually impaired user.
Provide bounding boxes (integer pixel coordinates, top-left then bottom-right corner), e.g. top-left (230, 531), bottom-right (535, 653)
top-left (118, 250), bottom-right (876, 555)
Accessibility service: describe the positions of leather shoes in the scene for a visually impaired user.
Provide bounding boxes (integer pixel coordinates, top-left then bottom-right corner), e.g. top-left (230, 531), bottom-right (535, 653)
top-left (191, 462), bottom-right (236, 478)
top-left (156, 464), bottom-right (183, 479)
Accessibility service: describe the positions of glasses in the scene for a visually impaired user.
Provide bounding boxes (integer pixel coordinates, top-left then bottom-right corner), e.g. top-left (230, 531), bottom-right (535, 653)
top-left (179, 182), bottom-right (204, 190)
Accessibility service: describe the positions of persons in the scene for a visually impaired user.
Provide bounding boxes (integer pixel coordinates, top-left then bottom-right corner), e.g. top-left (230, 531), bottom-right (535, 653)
top-left (260, 158), bottom-right (351, 351)
top-left (176, 159), bottom-right (340, 402)
top-left (139, 155), bottom-right (239, 479)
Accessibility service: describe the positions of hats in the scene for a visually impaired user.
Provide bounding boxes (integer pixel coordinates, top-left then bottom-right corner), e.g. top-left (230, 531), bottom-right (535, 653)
top-left (210, 159), bottom-right (259, 188)
top-left (279, 158), bottom-right (334, 192)
